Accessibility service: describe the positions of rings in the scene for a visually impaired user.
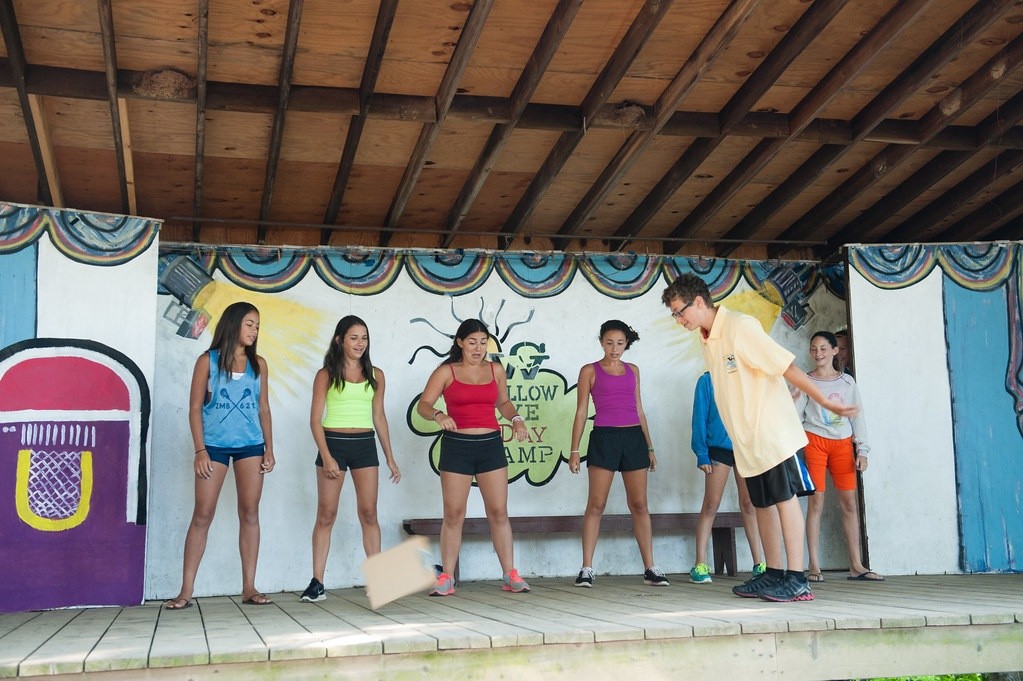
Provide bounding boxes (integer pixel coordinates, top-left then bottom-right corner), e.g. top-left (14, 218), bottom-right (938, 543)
top-left (654, 466), bottom-right (656, 467)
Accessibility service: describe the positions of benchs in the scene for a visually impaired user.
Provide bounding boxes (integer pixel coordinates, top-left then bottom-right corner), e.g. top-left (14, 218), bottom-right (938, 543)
top-left (402, 512), bottom-right (747, 588)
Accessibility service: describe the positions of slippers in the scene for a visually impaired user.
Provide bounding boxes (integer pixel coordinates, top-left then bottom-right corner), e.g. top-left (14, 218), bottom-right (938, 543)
top-left (808, 573), bottom-right (824, 582)
top-left (165, 598), bottom-right (193, 610)
top-left (847, 571), bottom-right (885, 581)
top-left (242, 594), bottom-right (273, 605)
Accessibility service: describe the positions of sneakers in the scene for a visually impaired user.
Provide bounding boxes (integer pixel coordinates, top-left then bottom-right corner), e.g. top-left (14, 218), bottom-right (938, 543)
top-left (428, 573), bottom-right (456, 596)
top-left (732, 572), bottom-right (784, 598)
top-left (502, 568), bottom-right (531, 593)
top-left (643, 566), bottom-right (670, 586)
top-left (574, 567), bottom-right (596, 588)
top-left (299, 577), bottom-right (327, 602)
top-left (688, 563), bottom-right (714, 583)
top-left (752, 562), bottom-right (766, 577)
top-left (757, 574), bottom-right (814, 601)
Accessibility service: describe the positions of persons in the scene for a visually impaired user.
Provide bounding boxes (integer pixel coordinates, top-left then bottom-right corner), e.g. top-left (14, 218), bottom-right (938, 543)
top-left (569, 320), bottom-right (670, 587)
top-left (663, 276), bottom-right (861, 602)
top-left (834, 330), bottom-right (852, 376)
top-left (165, 301), bottom-right (276, 610)
top-left (300, 315), bottom-right (402, 601)
top-left (791, 331), bottom-right (885, 583)
top-left (689, 371), bottom-right (765, 584)
top-left (417, 319), bottom-right (531, 595)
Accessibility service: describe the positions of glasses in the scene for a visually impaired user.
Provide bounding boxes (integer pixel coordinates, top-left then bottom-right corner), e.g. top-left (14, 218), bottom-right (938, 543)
top-left (672, 302), bottom-right (693, 319)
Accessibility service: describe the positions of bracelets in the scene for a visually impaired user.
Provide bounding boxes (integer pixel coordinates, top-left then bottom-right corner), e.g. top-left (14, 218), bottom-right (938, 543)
top-left (648, 448), bottom-right (654, 453)
top-left (511, 415), bottom-right (520, 419)
top-left (570, 451), bottom-right (578, 452)
top-left (195, 449), bottom-right (206, 454)
top-left (433, 411), bottom-right (443, 420)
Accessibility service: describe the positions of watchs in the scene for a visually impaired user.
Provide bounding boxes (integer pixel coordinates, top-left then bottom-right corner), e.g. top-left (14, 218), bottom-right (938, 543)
top-left (511, 416), bottom-right (523, 423)
